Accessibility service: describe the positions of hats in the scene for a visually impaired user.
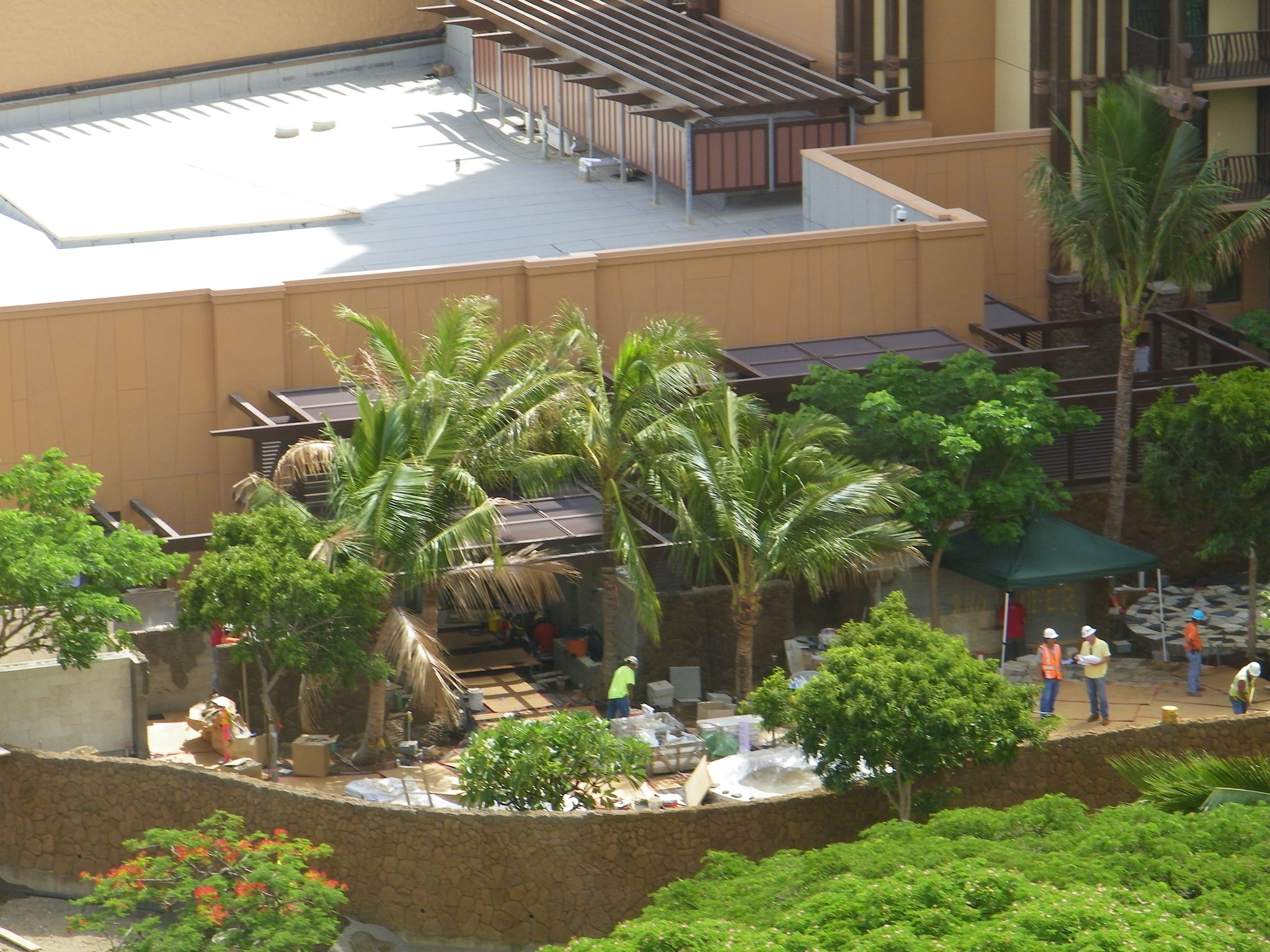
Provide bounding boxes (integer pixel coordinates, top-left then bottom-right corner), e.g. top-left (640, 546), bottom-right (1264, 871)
top-left (1081, 626), bottom-right (1097, 638)
top-left (1044, 628), bottom-right (1059, 638)
top-left (624, 656), bottom-right (639, 668)
top-left (1191, 610), bottom-right (1205, 620)
top-left (1248, 662), bottom-right (1261, 677)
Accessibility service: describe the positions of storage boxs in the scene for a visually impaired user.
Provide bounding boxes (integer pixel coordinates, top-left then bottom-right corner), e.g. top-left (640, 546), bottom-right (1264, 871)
top-left (696, 701), bottom-right (733, 720)
top-left (292, 734), bottom-right (342, 777)
top-left (184, 716), bottom-right (269, 779)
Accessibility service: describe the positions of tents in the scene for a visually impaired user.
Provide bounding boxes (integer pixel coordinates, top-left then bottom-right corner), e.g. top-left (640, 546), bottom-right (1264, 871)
top-left (922, 509), bottom-right (1168, 684)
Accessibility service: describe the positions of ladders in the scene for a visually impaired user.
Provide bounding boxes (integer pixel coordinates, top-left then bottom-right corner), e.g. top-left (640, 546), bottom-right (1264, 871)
top-left (396, 760), bottom-right (433, 808)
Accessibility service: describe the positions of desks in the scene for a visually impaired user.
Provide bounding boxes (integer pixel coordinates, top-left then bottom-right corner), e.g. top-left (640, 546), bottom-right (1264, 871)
top-left (610, 712), bottom-right (706, 775)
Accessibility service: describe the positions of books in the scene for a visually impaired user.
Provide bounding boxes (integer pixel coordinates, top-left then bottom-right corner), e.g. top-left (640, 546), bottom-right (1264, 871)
top-left (1073, 654), bottom-right (1101, 667)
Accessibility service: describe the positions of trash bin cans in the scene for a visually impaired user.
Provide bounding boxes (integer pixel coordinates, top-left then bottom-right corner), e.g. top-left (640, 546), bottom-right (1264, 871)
top-left (467, 688), bottom-right (484, 711)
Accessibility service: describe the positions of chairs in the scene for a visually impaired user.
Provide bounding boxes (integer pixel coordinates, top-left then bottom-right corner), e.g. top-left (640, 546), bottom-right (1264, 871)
top-left (670, 666), bottom-right (702, 722)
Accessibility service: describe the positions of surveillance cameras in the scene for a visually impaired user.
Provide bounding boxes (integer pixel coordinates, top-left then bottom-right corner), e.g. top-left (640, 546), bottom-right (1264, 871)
top-left (896, 210), bottom-right (908, 221)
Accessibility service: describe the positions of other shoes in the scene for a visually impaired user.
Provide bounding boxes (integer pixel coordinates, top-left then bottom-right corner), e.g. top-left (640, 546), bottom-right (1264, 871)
top-left (1102, 719), bottom-right (1109, 726)
top-left (1188, 692), bottom-right (1202, 697)
top-left (1197, 687), bottom-right (1207, 691)
top-left (1088, 715), bottom-right (1100, 722)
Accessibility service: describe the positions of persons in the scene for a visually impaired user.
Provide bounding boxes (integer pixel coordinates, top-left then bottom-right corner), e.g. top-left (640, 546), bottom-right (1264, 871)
top-left (1076, 626), bottom-right (1111, 725)
top-left (1185, 610), bottom-right (1207, 697)
top-left (1036, 628), bottom-right (1062, 722)
top-left (607, 655), bottom-right (638, 729)
top-left (531, 614), bottom-right (554, 655)
top-left (1228, 661), bottom-right (1261, 714)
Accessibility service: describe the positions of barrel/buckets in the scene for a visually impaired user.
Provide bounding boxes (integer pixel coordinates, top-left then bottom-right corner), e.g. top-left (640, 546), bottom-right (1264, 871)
top-left (468, 689), bottom-right (486, 710)
top-left (1161, 706), bottom-right (1177, 724)
